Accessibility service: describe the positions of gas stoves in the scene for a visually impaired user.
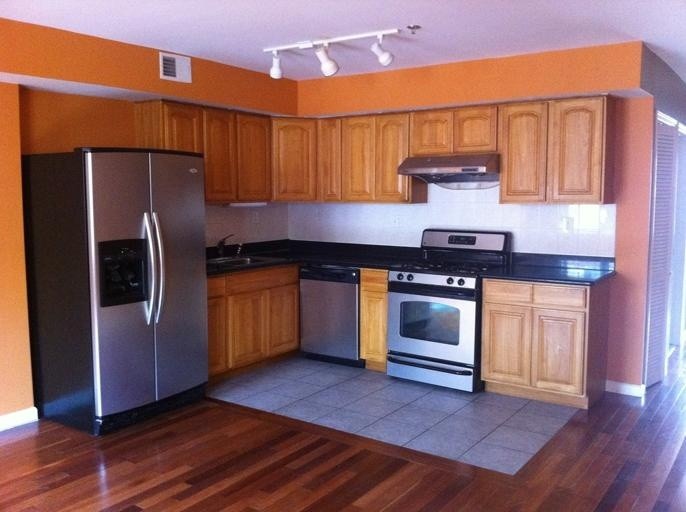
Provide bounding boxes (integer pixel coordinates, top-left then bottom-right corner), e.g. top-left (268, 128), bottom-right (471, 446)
top-left (387, 251), bottom-right (505, 294)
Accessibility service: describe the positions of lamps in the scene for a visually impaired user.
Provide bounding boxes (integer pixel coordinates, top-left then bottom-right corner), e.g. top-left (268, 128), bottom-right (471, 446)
top-left (368, 31), bottom-right (396, 70)
top-left (313, 40), bottom-right (341, 78)
top-left (267, 48), bottom-right (283, 80)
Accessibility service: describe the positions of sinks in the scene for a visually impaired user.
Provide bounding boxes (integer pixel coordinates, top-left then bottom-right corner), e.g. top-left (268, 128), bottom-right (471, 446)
top-left (208, 257), bottom-right (287, 266)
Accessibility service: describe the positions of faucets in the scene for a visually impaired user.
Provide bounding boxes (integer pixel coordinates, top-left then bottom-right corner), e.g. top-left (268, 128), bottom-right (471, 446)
top-left (218, 233), bottom-right (235, 258)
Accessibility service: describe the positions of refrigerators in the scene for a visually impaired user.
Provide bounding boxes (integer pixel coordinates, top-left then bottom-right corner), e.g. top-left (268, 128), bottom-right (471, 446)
top-left (22, 145), bottom-right (211, 436)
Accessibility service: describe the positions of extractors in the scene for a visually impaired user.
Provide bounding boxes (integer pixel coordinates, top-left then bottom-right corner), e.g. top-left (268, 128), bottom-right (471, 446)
top-left (396, 153), bottom-right (500, 191)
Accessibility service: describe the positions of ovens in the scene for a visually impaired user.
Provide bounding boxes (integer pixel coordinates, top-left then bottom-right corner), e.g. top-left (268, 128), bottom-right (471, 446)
top-left (386, 281), bottom-right (486, 395)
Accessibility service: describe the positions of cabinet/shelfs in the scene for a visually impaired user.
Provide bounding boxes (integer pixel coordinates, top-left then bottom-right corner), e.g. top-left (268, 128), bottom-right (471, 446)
top-left (206, 251), bottom-right (300, 412)
top-left (359, 267), bottom-right (389, 371)
top-left (134, 94), bottom-right (618, 208)
top-left (482, 276), bottom-right (612, 410)
